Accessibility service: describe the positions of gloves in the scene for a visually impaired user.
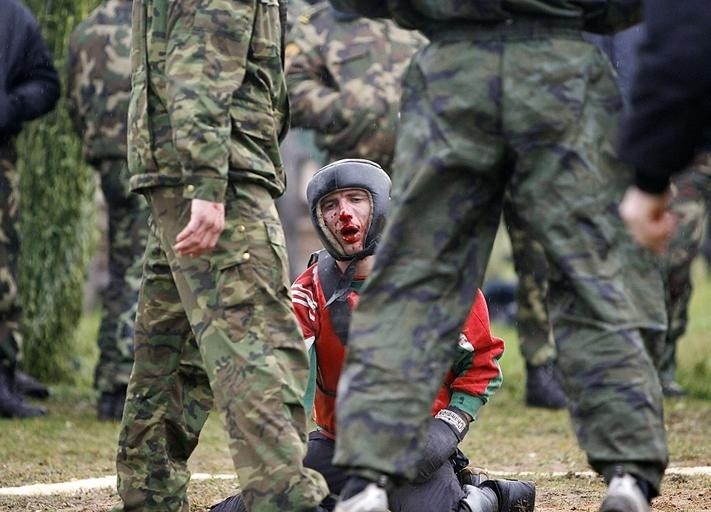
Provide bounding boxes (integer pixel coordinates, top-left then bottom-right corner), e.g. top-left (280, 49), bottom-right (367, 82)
top-left (393, 406), bottom-right (470, 486)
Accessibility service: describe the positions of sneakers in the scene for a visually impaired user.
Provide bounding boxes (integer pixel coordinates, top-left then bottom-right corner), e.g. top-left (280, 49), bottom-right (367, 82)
top-left (662, 378), bottom-right (689, 397)
top-left (0, 369), bottom-right (124, 422)
top-left (598, 472), bottom-right (652, 512)
top-left (456, 465), bottom-right (536, 512)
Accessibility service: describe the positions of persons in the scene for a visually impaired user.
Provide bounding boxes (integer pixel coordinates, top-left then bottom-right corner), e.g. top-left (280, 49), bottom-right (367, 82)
top-left (617, 5), bottom-right (711, 257)
top-left (210, 154), bottom-right (538, 512)
top-left (587, 26), bottom-right (711, 398)
top-left (61, 1), bottom-right (157, 424)
top-left (281, 1), bottom-right (433, 191)
top-left (113, 0), bottom-right (341, 512)
top-left (502, 208), bottom-right (573, 410)
top-left (326, 0), bottom-right (672, 511)
top-left (0, 2), bottom-right (62, 418)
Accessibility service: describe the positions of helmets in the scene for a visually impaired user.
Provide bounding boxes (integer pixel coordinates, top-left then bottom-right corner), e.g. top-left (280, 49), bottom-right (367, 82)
top-left (306, 158), bottom-right (392, 262)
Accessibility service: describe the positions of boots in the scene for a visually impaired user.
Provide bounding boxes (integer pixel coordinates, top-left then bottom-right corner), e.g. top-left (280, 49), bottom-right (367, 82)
top-left (526, 362), bottom-right (570, 410)
